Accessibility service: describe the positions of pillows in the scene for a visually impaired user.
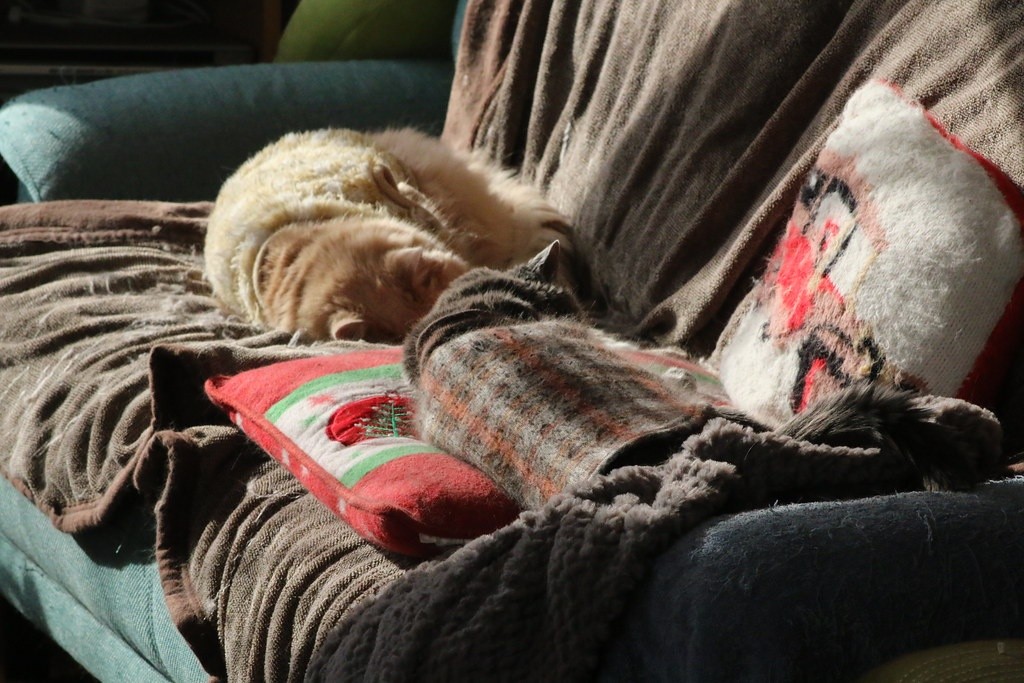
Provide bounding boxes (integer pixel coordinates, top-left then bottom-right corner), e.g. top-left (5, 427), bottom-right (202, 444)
top-left (707, 73), bottom-right (1024, 433)
top-left (204, 345), bottom-right (523, 560)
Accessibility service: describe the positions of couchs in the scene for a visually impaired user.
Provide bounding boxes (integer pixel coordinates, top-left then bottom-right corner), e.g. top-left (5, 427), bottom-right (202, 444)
top-left (1, 0), bottom-right (1024, 683)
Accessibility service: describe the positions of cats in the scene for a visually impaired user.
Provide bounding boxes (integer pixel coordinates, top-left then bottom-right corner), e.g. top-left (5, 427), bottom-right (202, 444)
top-left (401, 239), bottom-right (944, 514)
top-left (202, 124), bottom-right (579, 342)
